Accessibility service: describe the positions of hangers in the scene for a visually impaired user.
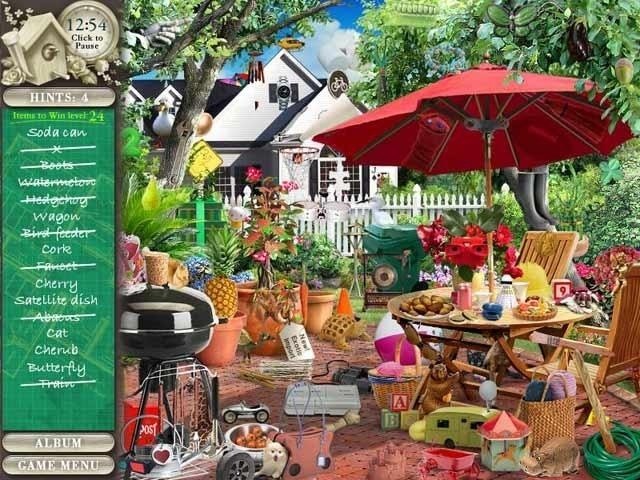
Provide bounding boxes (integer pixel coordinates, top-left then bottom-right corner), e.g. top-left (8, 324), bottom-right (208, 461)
top-left (387, 287), bottom-right (594, 411)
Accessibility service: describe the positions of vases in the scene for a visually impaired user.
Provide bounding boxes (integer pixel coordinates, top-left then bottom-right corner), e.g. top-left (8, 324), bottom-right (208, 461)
top-left (232, 281), bottom-right (338, 356)
top-left (196, 310), bottom-right (248, 367)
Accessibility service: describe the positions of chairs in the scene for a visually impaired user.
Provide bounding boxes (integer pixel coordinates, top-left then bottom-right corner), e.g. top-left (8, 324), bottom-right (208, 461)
top-left (443, 231), bottom-right (640, 454)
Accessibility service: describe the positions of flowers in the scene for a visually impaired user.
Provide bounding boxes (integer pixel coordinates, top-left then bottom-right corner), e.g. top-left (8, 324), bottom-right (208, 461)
top-left (230, 166), bottom-right (306, 289)
